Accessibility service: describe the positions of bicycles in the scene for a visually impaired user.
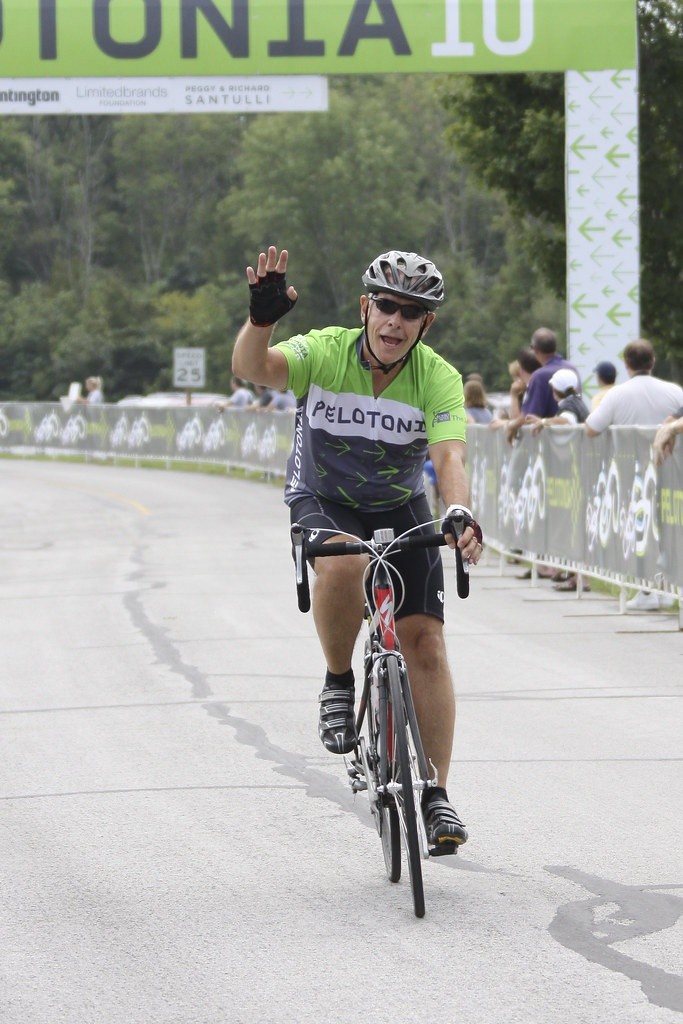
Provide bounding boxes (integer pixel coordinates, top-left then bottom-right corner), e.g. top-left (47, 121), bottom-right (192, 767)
top-left (289, 508), bottom-right (471, 919)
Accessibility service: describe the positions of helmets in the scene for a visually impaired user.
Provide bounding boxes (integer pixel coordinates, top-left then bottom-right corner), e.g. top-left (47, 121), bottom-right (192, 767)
top-left (362, 249), bottom-right (445, 312)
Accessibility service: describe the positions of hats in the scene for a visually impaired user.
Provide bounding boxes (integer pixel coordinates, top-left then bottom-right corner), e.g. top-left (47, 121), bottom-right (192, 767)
top-left (548, 367), bottom-right (580, 394)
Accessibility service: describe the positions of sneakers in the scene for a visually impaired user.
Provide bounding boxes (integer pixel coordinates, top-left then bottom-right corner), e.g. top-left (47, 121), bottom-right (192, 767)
top-left (420, 793), bottom-right (470, 845)
top-left (318, 682), bottom-right (359, 754)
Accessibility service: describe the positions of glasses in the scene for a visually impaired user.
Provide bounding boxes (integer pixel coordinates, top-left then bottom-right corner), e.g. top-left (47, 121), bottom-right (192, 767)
top-left (369, 297), bottom-right (426, 320)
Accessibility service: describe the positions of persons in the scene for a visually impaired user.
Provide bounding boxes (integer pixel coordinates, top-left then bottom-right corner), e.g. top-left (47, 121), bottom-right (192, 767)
top-left (423, 325), bottom-right (683, 611)
top-left (214, 375), bottom-right (298, 480)
top-left (233, 245), bottom-right (483, 841)
top-left (77, 376), bottom-right (103, 404)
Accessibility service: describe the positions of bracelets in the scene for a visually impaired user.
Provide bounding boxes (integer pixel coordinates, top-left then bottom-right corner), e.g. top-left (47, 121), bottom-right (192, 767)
top-left (542, 419), bottom-right (549, 425)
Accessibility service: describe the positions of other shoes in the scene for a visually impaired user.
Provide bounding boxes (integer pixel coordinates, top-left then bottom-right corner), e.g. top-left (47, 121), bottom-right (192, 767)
top-left (496, 549), bottom-right (668, 610)
